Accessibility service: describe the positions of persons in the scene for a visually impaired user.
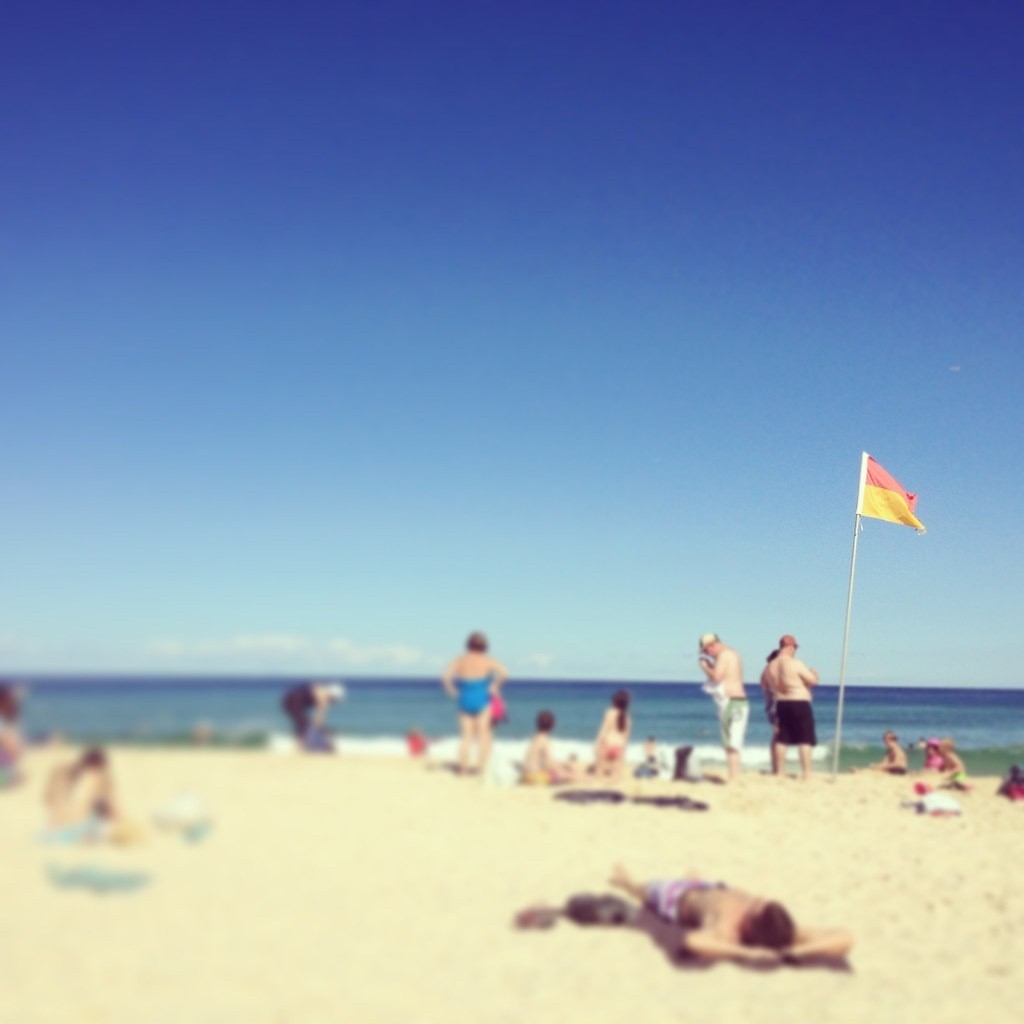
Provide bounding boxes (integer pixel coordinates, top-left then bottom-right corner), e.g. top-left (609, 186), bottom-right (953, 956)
top-left (700, 635), bottom-right (749, 779)
top-left (761, 635), bottom-right (822, 781)
top-left (995, 765), bottom-right (1024, 802)
top-left (0, 634), bottom-right (663, 844)
top-left (852, 730), bottom-right (968, 791)
top-left (610, 867), bottom-right (852, 963)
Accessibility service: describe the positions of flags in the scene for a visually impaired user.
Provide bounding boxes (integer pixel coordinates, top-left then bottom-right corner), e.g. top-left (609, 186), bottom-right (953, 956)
top-left (857, 452), bottom-right (924, 530)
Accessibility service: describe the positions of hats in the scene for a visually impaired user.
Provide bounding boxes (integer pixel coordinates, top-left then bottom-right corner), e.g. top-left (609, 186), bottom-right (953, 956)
top-left (700, 633), bottom-right (716, 653)
top-left (781, 636), bottom-right (797, 648)
